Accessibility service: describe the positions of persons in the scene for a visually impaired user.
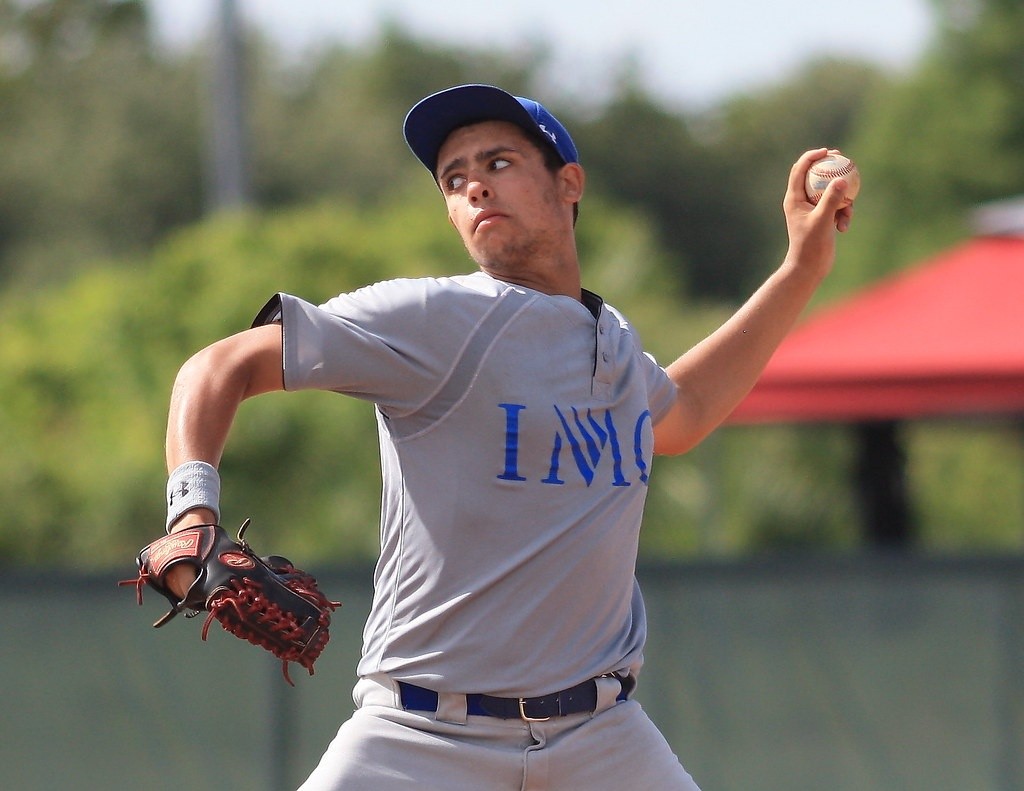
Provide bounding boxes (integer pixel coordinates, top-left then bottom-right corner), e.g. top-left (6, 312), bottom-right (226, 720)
top-left (132, 84), bottom-right (851, 791)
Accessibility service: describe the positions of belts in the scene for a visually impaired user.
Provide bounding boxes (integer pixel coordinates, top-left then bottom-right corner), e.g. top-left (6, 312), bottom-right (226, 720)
top-left (398, 671), bottom-right (628, 722)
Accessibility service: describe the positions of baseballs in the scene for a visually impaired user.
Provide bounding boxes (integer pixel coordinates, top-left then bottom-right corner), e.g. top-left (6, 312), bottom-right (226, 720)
top-left (805, 154), bottom-right (860, 210)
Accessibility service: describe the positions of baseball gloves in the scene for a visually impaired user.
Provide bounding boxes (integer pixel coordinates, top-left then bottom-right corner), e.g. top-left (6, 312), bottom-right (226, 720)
top-left (137, 524), bottom-right (334, 668)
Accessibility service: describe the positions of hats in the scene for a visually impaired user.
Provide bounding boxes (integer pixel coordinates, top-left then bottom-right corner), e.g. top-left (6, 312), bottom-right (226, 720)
top-left (403, 85), bottom-right (578, 229)
